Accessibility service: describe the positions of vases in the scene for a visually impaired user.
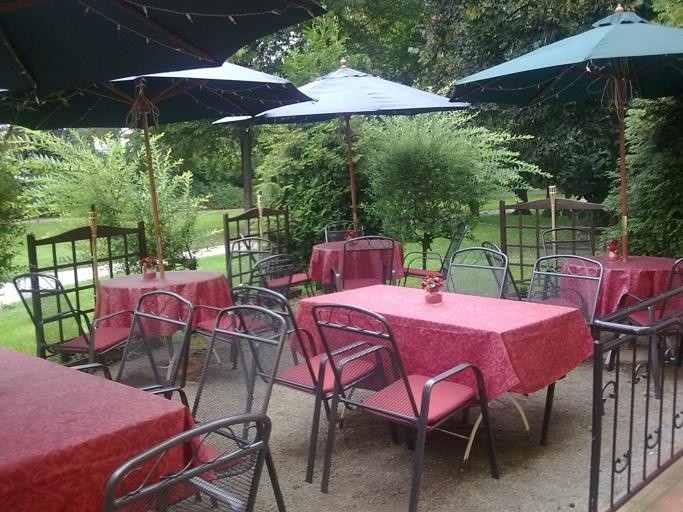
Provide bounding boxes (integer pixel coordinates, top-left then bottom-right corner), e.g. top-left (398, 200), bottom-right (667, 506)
top-left (143, 267), bottom-right (157, 281)
top-left (346, 236), bottom-right (352, 243)
top-left (425, 287), bottom-right (442, 303)
top-left (608, 250), bottom-right (617, 260)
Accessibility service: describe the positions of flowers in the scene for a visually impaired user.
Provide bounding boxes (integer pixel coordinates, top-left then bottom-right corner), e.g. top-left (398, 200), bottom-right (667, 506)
top-left (607, 240), bottom-right (618, 252)
top-left (345, 231), bottom-right (352, 236)
top-left (420, 274), bottom-right (444, 286)
top-left (140, 256), bottom-right (157, 267)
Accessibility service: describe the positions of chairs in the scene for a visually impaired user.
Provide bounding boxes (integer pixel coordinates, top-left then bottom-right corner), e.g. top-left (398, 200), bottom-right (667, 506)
top-left (12, 270), bottom-right (161, 396)
top-left (238, 231), bottom-right (313, 305)
top-left (226, 283), bottom-right (403, 485)
top-left (311, 301), bottom-right (502, 512)
top-left (65, 290), bottom-right (193, 417)
top-left (179, 254), bottom-right (298, 388)
top-left (607, 254), bottom-right (682, 399)
top-left (481, 239), bottom-right (526, 301)
top-left (96, 414), bottom-right (289, 512)
top-left (339, 235), bottom-right (395, 288)
top-left (227, 238), bottom-right (270, 310)
top-left (443, 246), bottom-right (508, 299)
top-left (324, 219), bottom-right (364, 242)
top-left (104, 305), bottom-right (287, 511)
top-left (397, 220), bottom-right (467, 288)
top-left (524, 254), bottom-right (608, 446)
top-left (540, 225), bottom-right (598, 301)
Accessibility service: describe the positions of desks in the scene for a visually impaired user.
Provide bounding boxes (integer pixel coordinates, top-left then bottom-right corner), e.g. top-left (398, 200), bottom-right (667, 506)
top-left (0, 344), bottom-right (201, 512)
top-left (95, 270), bottom-right (228, 368)
top-left (307, 236), bottom-right (405, 289)
top-left (294, 282), bottom-right (591, 448)
top-left (561, 256), bottom-right (681, 349)
